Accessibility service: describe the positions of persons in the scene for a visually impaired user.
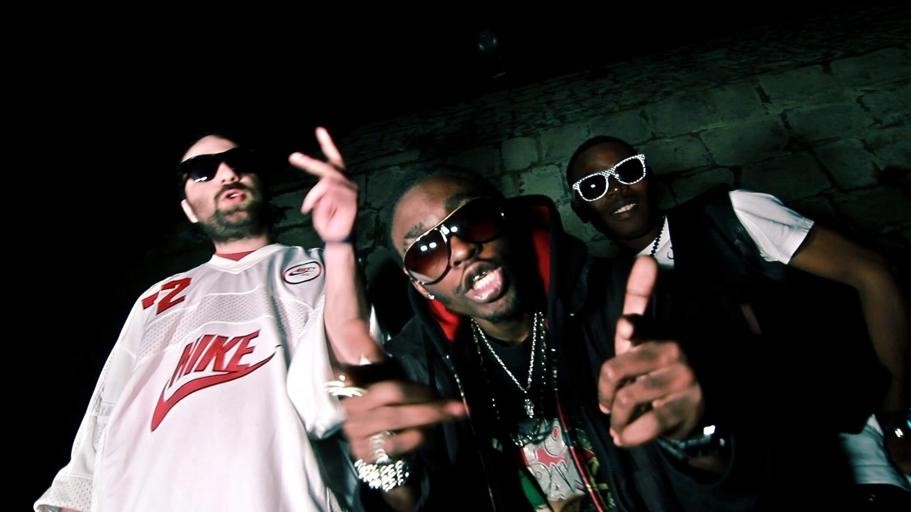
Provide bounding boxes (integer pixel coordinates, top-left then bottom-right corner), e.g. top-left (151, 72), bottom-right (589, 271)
top-left (32, 123), bottom-right (391, 512)
top-left (334, 157), bottom-right (761, 510)
top-left (567, 137), bottom-right (910, 511)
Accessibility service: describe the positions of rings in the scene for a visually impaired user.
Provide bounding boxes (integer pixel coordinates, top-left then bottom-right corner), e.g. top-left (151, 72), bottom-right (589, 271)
top-left (367, 430), bottom-right (398, 463)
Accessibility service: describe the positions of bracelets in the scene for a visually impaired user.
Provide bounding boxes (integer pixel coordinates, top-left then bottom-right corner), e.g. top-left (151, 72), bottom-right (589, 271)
top-left (880, 411), bottom-right (910, 438)
top-left (651, 421), bottom-right (731, 460)
top-left (348, 454), bottom-right (414, 492)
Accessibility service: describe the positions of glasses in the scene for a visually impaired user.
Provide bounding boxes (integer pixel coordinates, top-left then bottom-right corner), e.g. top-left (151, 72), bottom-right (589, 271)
top-left (404, 190), bottom-right (510, 286)
top-left (570, 150), bottom-right (651, 204)
top-left (180, 144), bottom-right (264, 194)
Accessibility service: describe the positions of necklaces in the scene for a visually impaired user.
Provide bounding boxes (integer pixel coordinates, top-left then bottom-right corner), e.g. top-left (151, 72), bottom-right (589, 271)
top-left (473, 310), bottom-right (547, 419)
top-left (644, 208), bottom-right (671, 263)
top-left (464, 306), bottom-right (550, 449)
